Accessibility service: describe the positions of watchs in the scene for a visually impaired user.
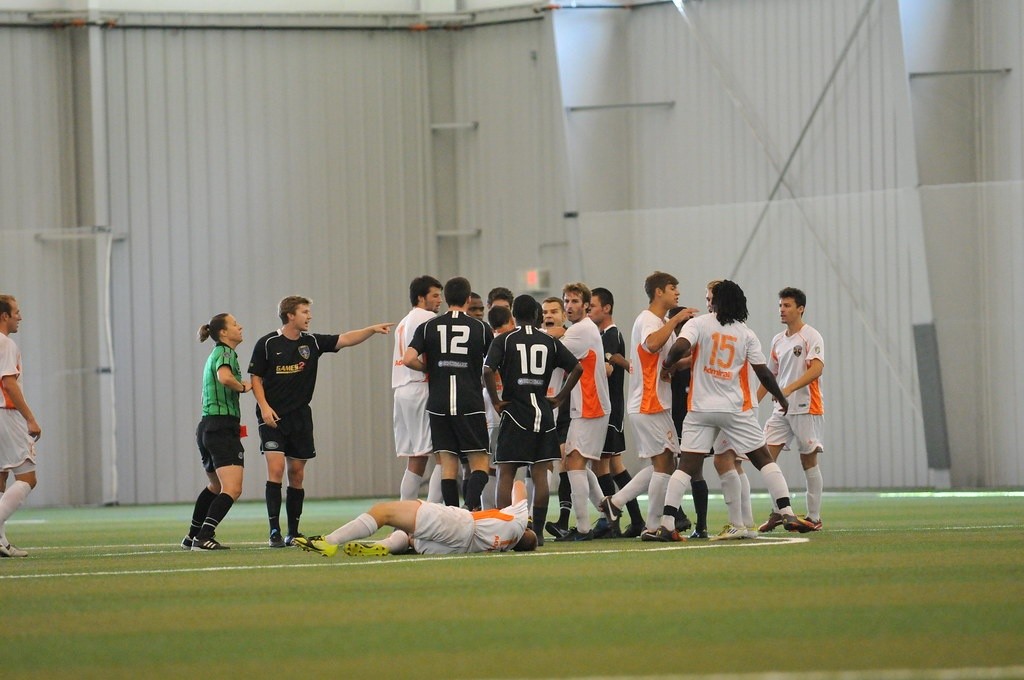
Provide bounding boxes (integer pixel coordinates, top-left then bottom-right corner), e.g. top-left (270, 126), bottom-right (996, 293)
top-left (241, 381), bottom-right (247, 394)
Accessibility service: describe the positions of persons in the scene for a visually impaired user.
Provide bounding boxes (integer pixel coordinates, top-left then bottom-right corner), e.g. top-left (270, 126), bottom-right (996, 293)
top-left (247, 296), bottom-right (395, 548)
top-left (181, 313), bottom-right (253, 552)
top-left (0, 295), bottom-right (41, 557)
top-left (291, 480), bottom-right (538, 558)
top-left (756, 286), bottom-right (834, 533)
top-left (392, 272), bottom-right (815, 546)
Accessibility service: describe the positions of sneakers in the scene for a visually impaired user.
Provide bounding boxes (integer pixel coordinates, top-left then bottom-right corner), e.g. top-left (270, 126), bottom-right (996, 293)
top-left (713, 524), bottom-right (748, 540)
top-left (292, 536), bottom-right (339, 558)
top-left (0, 542), bottom-right (11, 557)
top-left (555, 526), bottom-right (594, 541)
top-left (784, 517), bottom-right (822, 531)
top-left (269, 533), bottom-right (285, 547)
top-left (746, 523), bottom-right (758, 538)
top-left (642, 525), bottom-right (683, 542)
top-left (546, 522), bottom-right (570, 538)
top-left (343, 542), bottom-right (390, 556)
top-left (622, 521), bottom-right (645, 538)
top-left (191, 536), bottom-right (230, 551)
top-left (285, 532), bottom-right (305, 547)
top-left (688, 526), bottom-right (708, 539)
top-left (676, 518), bottom-right (691, 532)
top-left (758, 509), bottom-right (797, 533)
top-left (6, 544), bottom-right (28, 557)
top-left (180, 535), bottom-right (193, 550)
top-left (599, 495), bottom-right (620, 537)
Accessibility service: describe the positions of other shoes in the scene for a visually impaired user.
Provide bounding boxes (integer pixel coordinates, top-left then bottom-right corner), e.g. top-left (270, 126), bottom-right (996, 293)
top-left (593, 518), bottom-right (621, 539)
